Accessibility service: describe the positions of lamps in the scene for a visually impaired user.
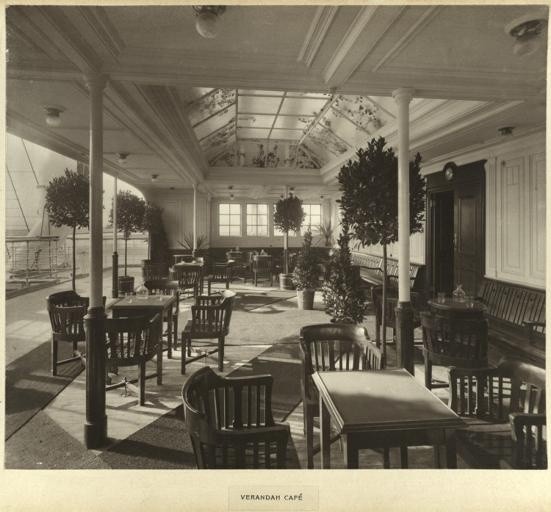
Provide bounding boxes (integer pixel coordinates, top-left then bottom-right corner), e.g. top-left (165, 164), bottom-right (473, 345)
top-left (114, 152), bottom-right (130, 167)
top-left (192, 5), bottom-right (225, 38)
top-left (510, 19), bottom-right (546, 56)
top-left (46, 105), bottom-right (66, 126)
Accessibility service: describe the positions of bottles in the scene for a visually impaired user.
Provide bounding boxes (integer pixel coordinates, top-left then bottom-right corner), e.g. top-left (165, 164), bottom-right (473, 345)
top-left (134, 281), bottom-right (148, 300)
top-left (452, 284), bottom-right (465, 298)
top-left (259, 249), bottom-right (266, 256)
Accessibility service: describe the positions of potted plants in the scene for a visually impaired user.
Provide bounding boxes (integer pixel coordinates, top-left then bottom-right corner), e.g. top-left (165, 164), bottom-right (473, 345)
top-left (274, 196), bottom-right (306, 290)
top-left (139, 200), bottom-right (163, 271)
top-left (109, 190), bottom-right (145, 295)
top-left (292, 231), bottom-right (314, 310)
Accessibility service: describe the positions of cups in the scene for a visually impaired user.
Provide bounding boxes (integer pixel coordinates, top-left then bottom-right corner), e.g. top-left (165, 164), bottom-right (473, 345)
top-left (124, 292), bottom-right (132, 303)
top-left (437, 292), bottom-right (444, 305)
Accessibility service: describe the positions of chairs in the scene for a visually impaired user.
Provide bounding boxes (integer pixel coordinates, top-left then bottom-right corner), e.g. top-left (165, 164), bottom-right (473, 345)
top-left (46, 251), bottom-right (546, 469)
